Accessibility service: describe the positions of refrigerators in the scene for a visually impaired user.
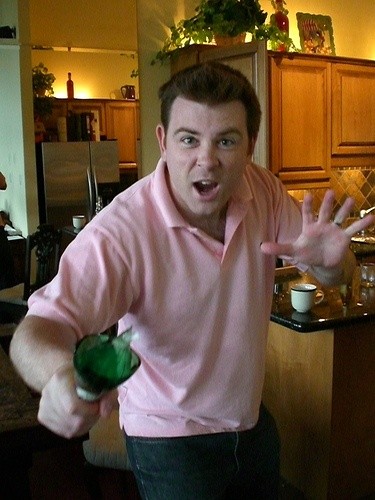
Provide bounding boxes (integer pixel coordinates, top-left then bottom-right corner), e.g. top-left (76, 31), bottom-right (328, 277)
top-left (35, 138), bottom-right (120, 229)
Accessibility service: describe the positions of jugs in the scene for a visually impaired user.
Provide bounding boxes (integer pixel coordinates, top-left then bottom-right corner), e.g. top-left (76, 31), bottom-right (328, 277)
top-left (121, 85), bottom-right (135, 99)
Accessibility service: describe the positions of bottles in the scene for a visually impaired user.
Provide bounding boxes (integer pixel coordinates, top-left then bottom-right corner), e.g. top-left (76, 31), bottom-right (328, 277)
top-left (66, 72), bottom-right (74, 99)
top-left (269, 0), bottom-right (289, 52)
top-left (72, 325), bottom-right (141, 405)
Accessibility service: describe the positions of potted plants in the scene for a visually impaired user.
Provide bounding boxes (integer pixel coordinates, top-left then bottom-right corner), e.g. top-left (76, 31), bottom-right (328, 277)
top-left (32, 62), bottom-right (56, 97)
top-left (150, 0), bottom-right (300, 67)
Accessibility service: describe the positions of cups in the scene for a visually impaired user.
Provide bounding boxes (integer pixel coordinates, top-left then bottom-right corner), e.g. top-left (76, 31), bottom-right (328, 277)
top-left (72, 215), bottom-right (86, 234)
top-left (290, 283), bottom-right (325, 313)
top-left (360, 262), bottom-right (375, 288)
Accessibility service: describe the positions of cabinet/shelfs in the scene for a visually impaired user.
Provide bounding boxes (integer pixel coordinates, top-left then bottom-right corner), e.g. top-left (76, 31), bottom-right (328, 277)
top-left (267, 49), bottom-right (375, 189)
top-left (34, 97), bottom-right (139, 173)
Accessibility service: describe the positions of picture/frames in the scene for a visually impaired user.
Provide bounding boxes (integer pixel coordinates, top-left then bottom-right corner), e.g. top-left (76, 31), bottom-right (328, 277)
top-left (296, 12), bottom-right (336, 57)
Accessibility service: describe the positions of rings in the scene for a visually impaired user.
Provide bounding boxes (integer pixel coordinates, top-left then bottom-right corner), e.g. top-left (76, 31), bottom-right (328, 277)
top-left (336, 222), bottom-right (341, 225)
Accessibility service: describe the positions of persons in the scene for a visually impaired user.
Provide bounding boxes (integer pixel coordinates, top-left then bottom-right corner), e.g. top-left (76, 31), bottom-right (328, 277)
top-left (0, 172), bottom-right (7, 240)
top-left (9, 61), bottom-right (375, 499)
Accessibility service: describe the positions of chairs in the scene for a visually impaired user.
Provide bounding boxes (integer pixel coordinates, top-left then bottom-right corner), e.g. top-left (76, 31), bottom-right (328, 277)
top-left (0, 224), bottom-right (63, 299)
